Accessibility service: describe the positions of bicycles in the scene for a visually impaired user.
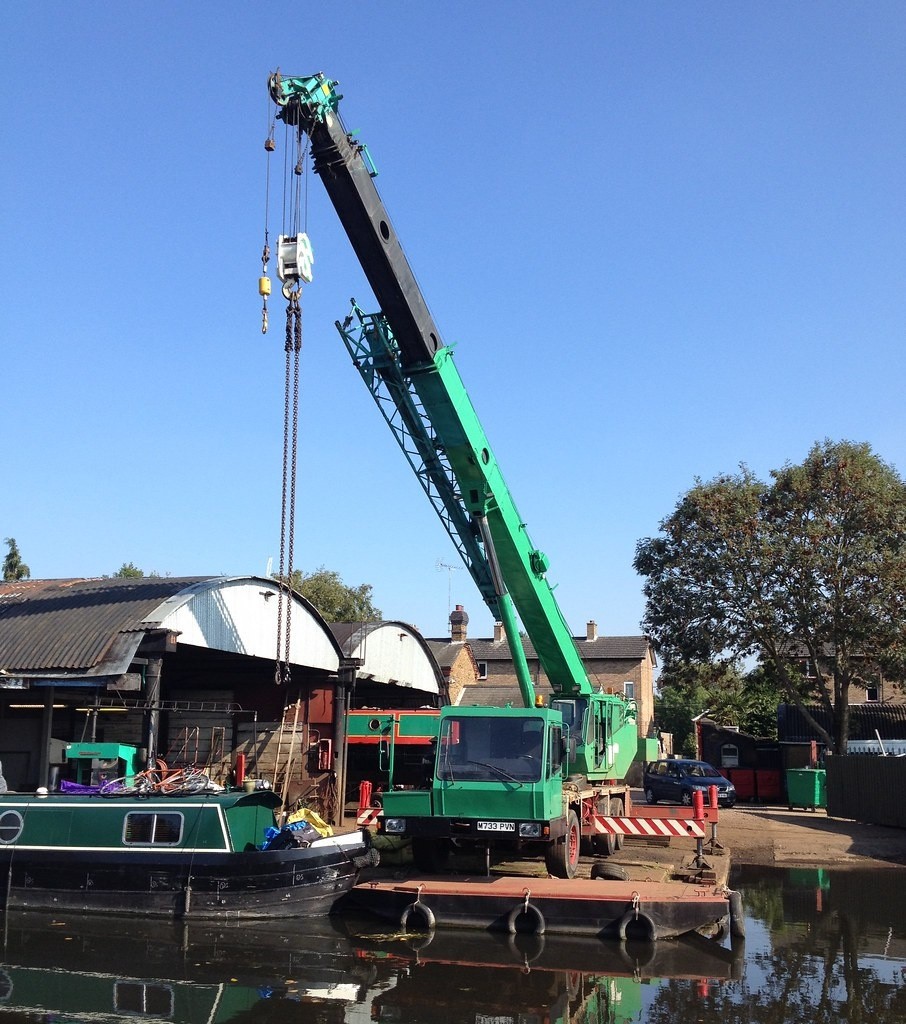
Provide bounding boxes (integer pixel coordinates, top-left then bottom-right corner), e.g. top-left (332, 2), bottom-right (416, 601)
top-left (99, 752), bottom-right (209, 798)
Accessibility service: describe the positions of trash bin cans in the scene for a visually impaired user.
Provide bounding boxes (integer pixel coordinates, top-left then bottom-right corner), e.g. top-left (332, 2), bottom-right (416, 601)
top-left (786, 768), bottom-right (828, 813)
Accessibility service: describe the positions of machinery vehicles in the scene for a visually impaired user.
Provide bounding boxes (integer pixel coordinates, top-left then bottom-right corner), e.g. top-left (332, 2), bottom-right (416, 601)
top-left (259, 73), bottom-right (726, 879)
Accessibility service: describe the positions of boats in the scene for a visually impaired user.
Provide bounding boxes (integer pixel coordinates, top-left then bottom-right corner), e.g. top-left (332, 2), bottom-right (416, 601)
top-left (352, 880), bottom-right (732, 940)
top-left (2, 793), bottom-right (381, 915)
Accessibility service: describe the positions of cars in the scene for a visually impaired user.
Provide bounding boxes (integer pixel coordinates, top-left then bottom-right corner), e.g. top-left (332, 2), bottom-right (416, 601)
top-left (643, 759), bottom-right (736, 809)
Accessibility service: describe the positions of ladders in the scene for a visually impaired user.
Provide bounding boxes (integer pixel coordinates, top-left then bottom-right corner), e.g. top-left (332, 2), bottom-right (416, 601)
top-left (272, 688), bottom-right (302, 831)
top-left (209, 726), bottom-right (225, 789)
